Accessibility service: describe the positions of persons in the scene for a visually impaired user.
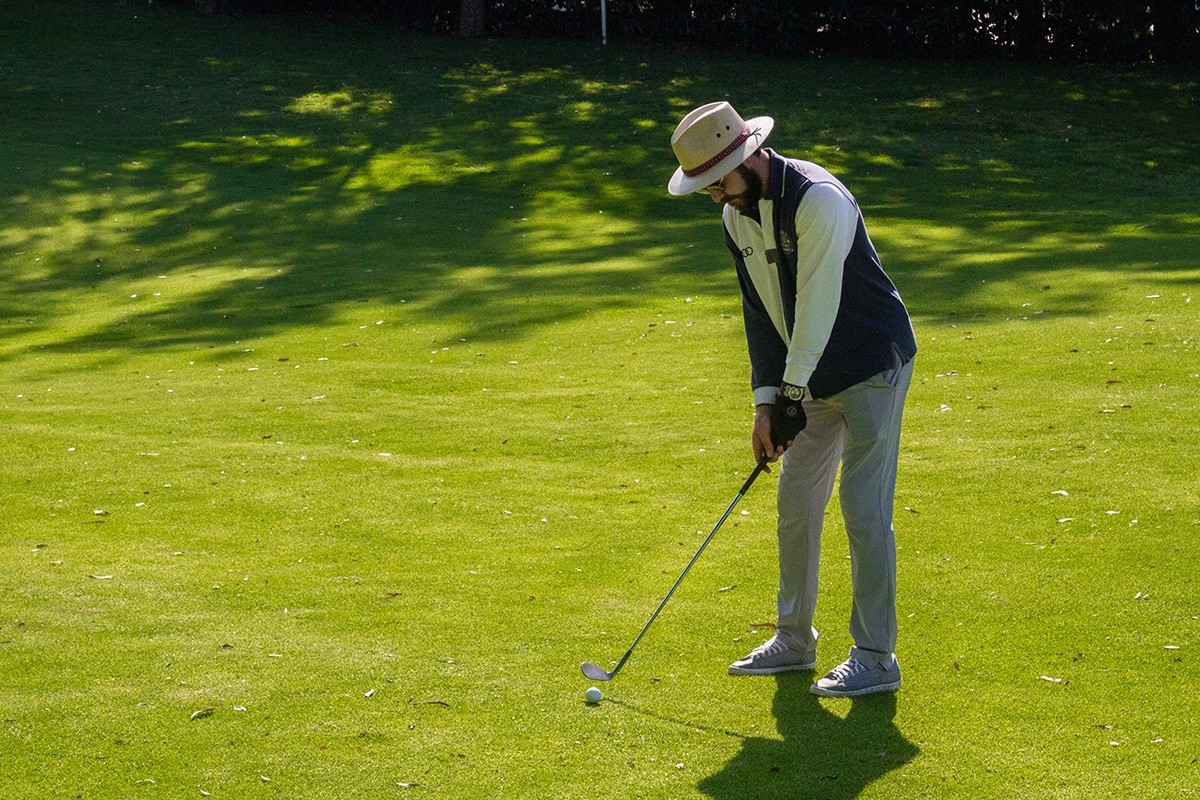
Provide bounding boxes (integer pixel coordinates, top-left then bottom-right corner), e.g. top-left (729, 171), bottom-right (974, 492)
top-left (668, 101), bottom-right (916, 698)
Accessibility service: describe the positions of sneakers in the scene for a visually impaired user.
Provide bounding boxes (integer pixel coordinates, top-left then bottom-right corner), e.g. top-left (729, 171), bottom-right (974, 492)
top-left (728, 635), bottom-right (816, 674)
top-left (810, 654), bottom-right (901, 697)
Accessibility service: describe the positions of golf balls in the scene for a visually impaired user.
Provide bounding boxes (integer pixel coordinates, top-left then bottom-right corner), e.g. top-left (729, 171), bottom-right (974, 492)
top-left (584, 687), bottom-right (601, 703)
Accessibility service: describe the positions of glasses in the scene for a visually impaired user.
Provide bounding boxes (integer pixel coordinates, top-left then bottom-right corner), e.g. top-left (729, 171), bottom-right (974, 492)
top-left (695, 177), bottom-right (724, 194)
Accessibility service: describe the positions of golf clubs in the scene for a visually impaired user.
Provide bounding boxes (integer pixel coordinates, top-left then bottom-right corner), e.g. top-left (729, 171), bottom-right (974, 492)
top-left (581, 454), bottom-right (774, 681)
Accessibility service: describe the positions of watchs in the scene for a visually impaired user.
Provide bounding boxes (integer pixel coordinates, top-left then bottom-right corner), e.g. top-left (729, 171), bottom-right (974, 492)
top-left (780, 381), bottom-right (805, 401)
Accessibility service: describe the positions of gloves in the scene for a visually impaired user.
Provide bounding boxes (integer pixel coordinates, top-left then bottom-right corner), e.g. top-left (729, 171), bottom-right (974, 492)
top-left (763, 393), bottom-right (807, 453)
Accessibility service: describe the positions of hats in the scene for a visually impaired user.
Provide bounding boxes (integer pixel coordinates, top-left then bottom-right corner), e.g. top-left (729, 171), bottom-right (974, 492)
top-left (668, 101), bottom-right (774, 196)
